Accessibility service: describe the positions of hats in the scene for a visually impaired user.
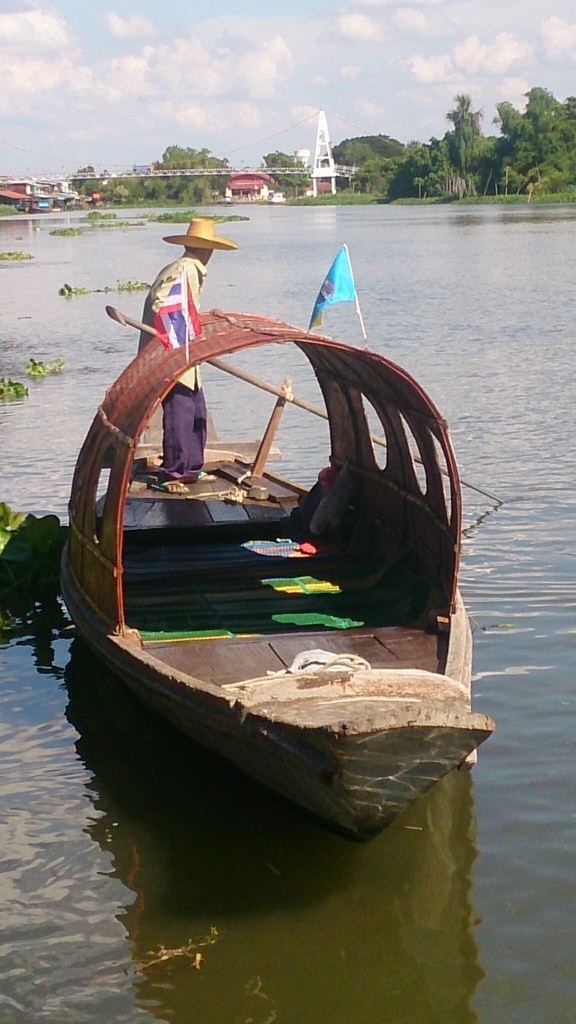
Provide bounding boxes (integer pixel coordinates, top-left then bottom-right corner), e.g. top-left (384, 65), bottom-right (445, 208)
top-left (162, 217), bottom-right (239, 250)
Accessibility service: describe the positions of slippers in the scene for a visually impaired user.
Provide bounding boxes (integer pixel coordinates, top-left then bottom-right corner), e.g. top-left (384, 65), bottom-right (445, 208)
top-left (150, 480), bottom-right (190, 493)
top-left (183, 471), bottom-right (216, 483)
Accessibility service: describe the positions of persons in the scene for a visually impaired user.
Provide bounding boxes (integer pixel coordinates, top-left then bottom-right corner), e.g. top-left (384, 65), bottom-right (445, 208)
top-left (138, 217), bottom-right (238, 494)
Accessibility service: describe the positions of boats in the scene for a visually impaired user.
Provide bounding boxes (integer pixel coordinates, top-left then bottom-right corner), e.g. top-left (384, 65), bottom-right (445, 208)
top-left (267, 191), bottom-right (287, 205)
top-left (58, 309), bottom-right (499, 842)
top-left (18, 199), bottom-right (51, 214)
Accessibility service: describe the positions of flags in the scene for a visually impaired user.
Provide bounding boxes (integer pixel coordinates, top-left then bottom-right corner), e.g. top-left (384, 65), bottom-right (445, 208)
top-left (153, 272), bottom-right (202, 350)
top-left (307, 247), bottom-right (356, 331)
